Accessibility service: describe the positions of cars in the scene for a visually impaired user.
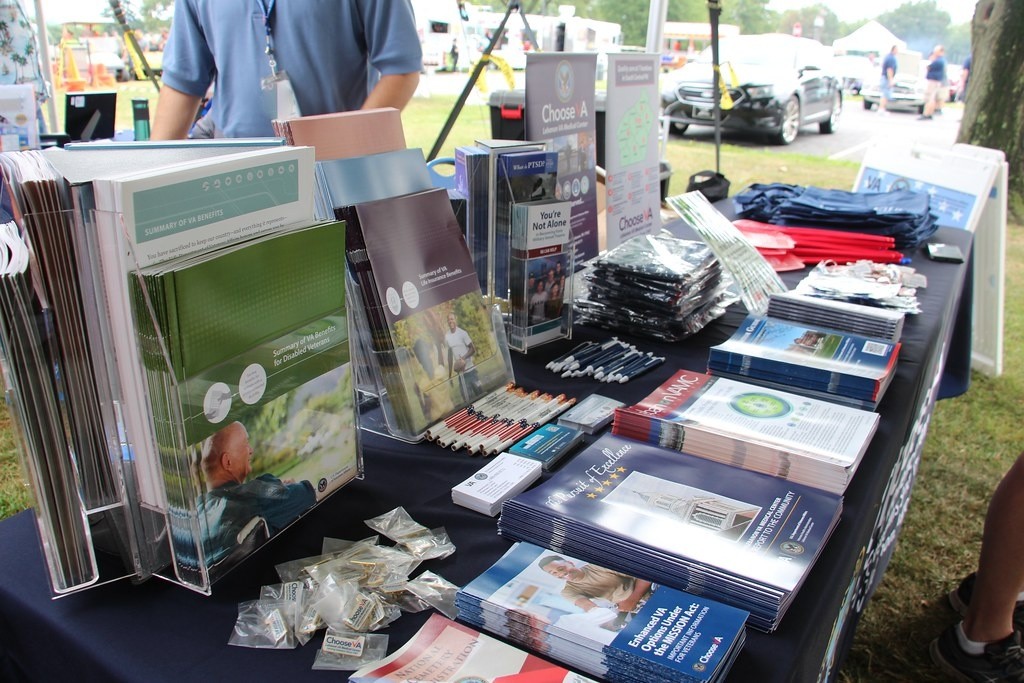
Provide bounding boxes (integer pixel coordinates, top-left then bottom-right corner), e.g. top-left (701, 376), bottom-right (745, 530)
top-left (659, 32), bottom-right (844, 144)
top-left (860, 50), bottom-right (965, 114)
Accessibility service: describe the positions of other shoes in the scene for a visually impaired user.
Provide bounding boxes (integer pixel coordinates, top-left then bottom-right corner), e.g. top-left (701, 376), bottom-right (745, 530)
top-left (916, 115), bottom-right (932, 121)
top-left (878, 110), bottom-right (889, 115)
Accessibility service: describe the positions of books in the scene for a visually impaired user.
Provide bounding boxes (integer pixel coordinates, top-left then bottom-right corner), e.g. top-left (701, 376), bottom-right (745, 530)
top-left (91, 143), bottom-right (318, 520)
top-left (130, 218), bottom-right (360, 589)
top-left (455, 136), bottom-right (559, 303)
top-left (612, 367), bottom-right (862, 497)
top-left (705, 315), bottom-right (903, 411)
top-left (767, 290), bottom-right (906, 345)
top-left (349, 614), bottom-right (601, 683)
top-left (452, 540), bottom-right (751, 683)
top-left (495, 430), bottom-right (845, 635)
top-left (513, 198), bottom-right (572, 349)
top-left (273, 106), bottom-right (455, 390)
top-left (334, 189), bottom-right (510, 435)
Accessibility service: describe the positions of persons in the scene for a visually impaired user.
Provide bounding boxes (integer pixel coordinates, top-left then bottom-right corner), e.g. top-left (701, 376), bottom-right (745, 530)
top-left (927, 449), bottom-right (1024, 683)
top-left (202, 421), bottom-right (315, 566)
top-left (954, 53), bottom-right (971, 102)
top-left (149, 0), bottom-right (425, 142)
top-left (916, 44), bottom-right (945, 120)
top-left (538, 555), bottom-right (653, 633)
top-left (876, 44), bottom-right (898, 115)
top-left (441, 310), bottom-right (484, 404)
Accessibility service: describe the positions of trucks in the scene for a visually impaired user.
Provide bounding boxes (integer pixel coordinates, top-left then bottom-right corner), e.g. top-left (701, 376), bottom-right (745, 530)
top-left (409, 0), bottom-right (622, 73)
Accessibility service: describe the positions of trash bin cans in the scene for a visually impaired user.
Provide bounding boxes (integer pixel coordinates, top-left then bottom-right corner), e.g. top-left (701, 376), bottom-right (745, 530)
top-left (486, 89), bottom-right (605, 169)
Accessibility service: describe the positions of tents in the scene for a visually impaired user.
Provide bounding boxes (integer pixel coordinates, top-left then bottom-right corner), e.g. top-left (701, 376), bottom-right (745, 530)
top-left (831, 19), bottom-right (907, 61)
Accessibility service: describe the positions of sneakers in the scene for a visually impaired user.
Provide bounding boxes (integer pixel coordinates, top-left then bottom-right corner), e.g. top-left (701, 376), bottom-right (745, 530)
top-left (928, 620), bottom-right (1024, 683)
top-left (949, 572), bottom-right (1024, 632)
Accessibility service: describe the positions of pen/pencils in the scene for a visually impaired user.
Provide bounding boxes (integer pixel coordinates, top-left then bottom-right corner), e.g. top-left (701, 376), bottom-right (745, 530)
top-left (424, 335), bottom-right (668, 458)
